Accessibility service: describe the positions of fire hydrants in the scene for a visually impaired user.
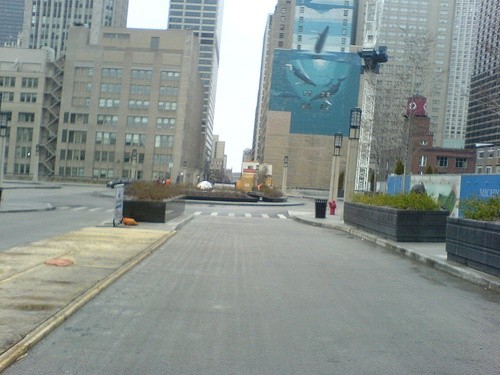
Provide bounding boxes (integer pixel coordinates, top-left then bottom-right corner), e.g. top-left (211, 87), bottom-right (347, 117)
top-left (329, 201), bottom-right (336, 215)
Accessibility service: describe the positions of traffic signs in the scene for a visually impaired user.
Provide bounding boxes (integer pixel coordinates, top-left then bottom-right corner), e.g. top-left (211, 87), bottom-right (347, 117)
top-left (344, 107), bottom-right (362, 202)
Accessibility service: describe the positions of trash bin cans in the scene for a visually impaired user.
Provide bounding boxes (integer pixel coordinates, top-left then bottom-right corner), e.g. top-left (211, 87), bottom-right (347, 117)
top-left (314, 198), bottom-right (328, 218)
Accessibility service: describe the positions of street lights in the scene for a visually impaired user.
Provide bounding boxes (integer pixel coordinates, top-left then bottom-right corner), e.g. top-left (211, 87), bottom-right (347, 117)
top-left (280, 156), bottom-right (288, 197)
top-left (328, 130), bottom-right (343, 201)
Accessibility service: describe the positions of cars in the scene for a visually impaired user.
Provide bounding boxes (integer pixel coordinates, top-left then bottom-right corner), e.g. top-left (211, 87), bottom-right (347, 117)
top-left (107, 177), bottom-right (133, 189)
